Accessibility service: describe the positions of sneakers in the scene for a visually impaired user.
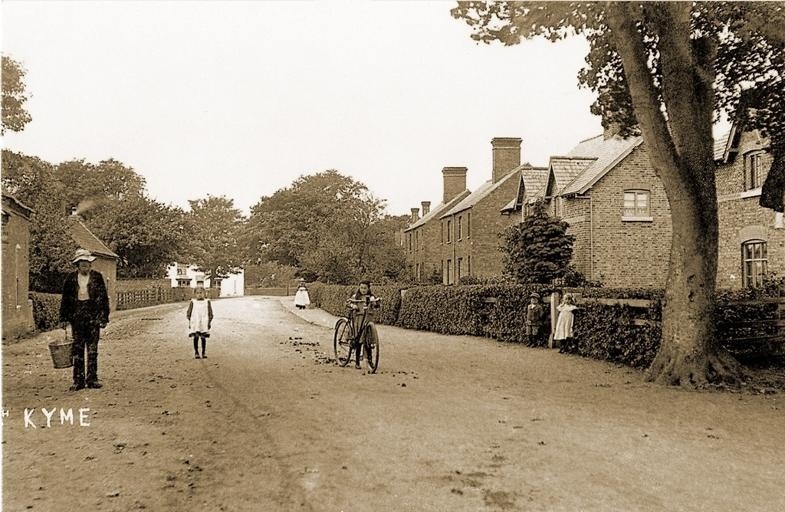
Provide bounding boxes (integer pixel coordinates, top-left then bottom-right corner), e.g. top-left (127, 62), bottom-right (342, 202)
top-left (68, 381), bottom-right (85, 391)
top-left (355, 364), bottom-right (361, 369)
top-left (87, 380), bottom-right (102, 388)
top-left (194, 353), bottom-right (207, 359)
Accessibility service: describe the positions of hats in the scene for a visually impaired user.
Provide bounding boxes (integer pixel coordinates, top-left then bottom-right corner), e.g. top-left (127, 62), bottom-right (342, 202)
top-left (71, 249), bottom-right (96, 264)
top-left (299, 277), bottom-right (306, 282)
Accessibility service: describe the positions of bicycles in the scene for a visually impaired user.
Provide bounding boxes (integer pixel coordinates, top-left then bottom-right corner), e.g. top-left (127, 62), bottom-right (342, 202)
top-left (332, 297), bottom-right (384, 376)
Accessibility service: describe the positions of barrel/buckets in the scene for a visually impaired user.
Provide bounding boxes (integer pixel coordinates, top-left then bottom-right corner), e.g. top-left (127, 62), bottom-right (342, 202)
top-left (48, 322), bottom-right (74, 369)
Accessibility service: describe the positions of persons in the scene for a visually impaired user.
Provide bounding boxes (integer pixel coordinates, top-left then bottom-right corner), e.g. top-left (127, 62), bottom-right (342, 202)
top-left (345, 280), bottom-right (381, 369)
top-left (186, 286), bottom-right (214, 359)
top-left (59, 247), bottom-right (111, 391)
top-left (525, 292), bottom-right (544, 348)
top-left (553, 293), bottom-right (579, 342)
top-left (294, 277), bottom-right (312, 310)
